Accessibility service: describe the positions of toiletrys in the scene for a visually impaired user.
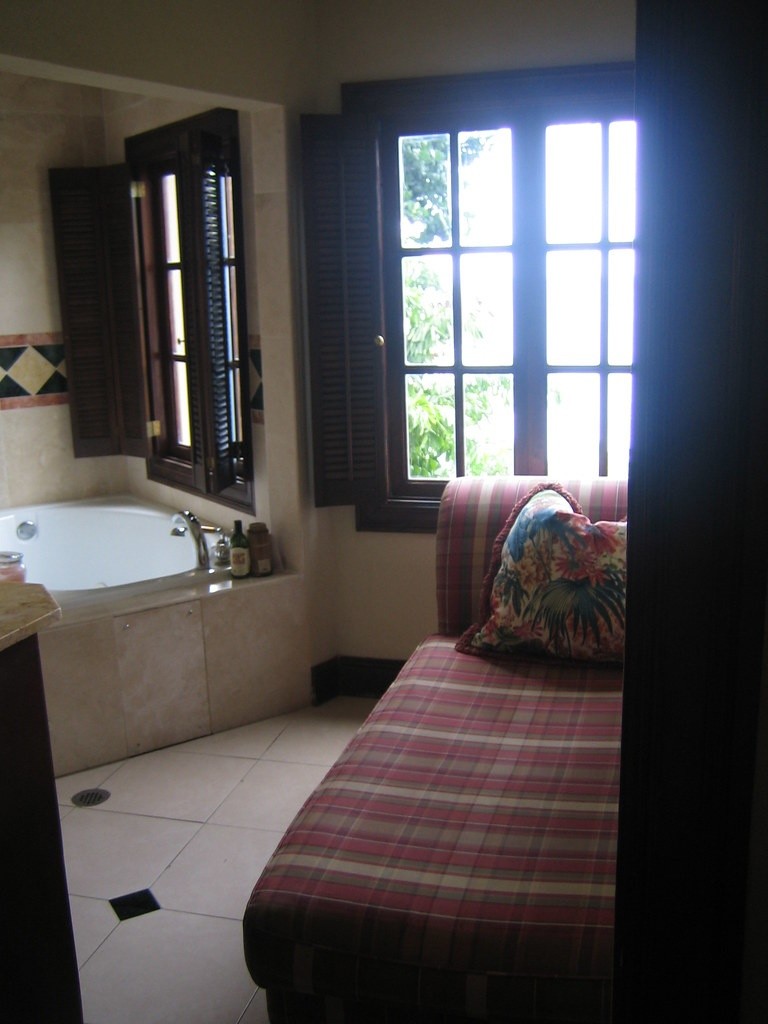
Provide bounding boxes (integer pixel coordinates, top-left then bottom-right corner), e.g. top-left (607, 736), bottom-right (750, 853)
top-left (0, 550), bottom-right (27, 583)
top-left (228, 517), bottom-right (270, 578)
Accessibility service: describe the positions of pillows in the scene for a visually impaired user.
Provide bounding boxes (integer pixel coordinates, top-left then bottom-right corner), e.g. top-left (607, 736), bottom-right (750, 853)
top-left (454, 482), bottom-right (626, 682)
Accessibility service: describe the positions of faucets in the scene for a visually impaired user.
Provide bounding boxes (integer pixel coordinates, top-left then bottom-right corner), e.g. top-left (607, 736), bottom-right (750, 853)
top-left (169, 509), bottom-right (210, 569)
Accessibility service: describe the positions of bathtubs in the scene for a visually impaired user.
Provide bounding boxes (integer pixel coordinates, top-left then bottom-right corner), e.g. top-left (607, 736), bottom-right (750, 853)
top-left (0, 499), bottom-right (243, 598)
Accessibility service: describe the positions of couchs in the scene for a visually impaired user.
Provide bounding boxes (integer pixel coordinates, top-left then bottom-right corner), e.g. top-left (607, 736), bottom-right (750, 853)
top-left (242, 477), bottom-right (630, 1024)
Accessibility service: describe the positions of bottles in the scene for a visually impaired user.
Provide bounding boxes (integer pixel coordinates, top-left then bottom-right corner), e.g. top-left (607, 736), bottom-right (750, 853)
top-left (247, 522), bottom-right (273, 577)
top-left (229, 520), bottom-right (251, 579)
top-left (0, 551), bottom-right (27, 583)
